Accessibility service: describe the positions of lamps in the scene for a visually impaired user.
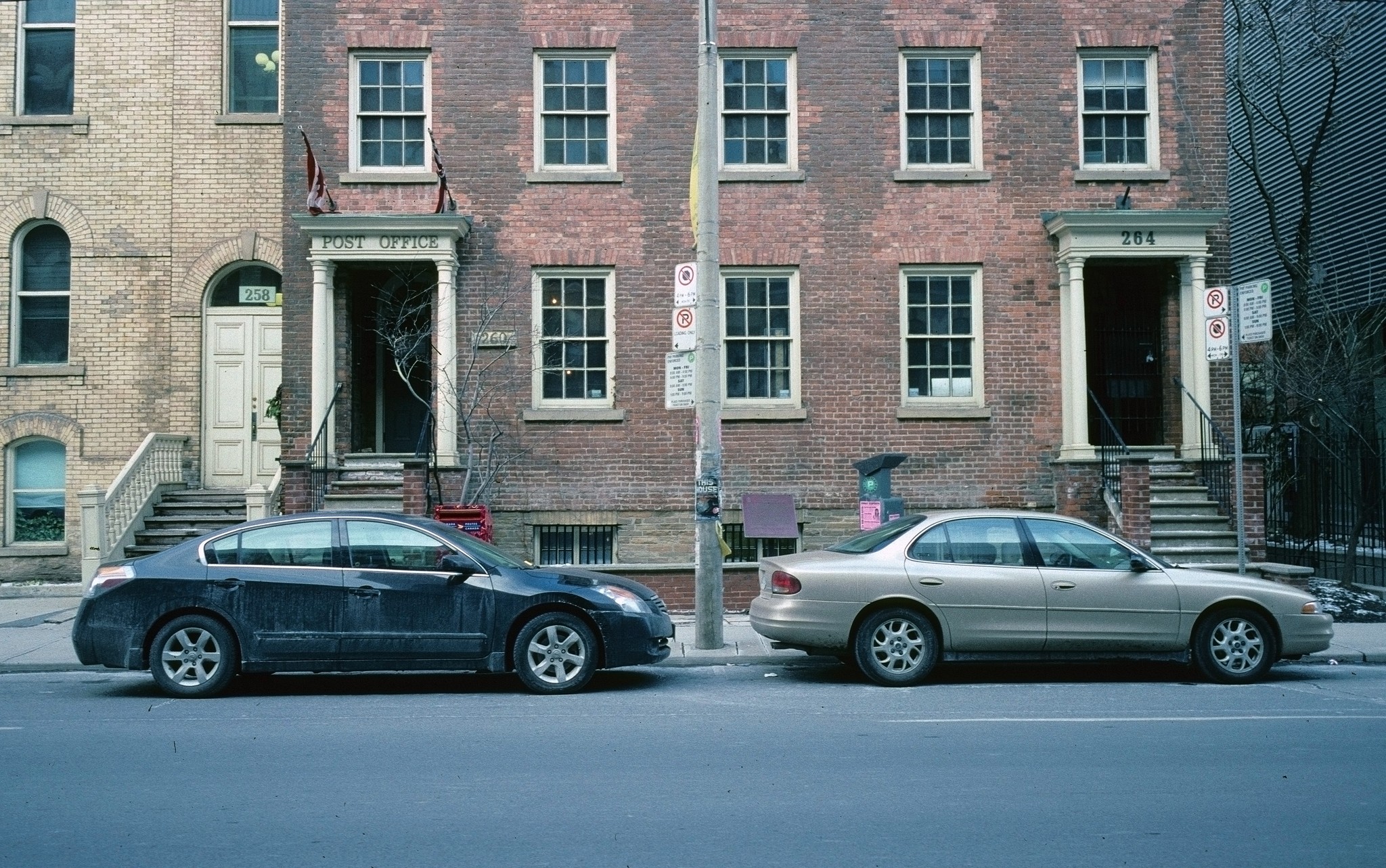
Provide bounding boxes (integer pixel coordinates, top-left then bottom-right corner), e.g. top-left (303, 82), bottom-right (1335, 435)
top-left (253, 48), bottom-right (279, 77)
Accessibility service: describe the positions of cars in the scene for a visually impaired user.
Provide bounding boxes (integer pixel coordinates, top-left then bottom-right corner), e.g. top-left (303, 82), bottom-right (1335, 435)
top-left (750, 509), bottom-right (1336, 688)
top-left (73, 514), bottom-right (676, 700)
